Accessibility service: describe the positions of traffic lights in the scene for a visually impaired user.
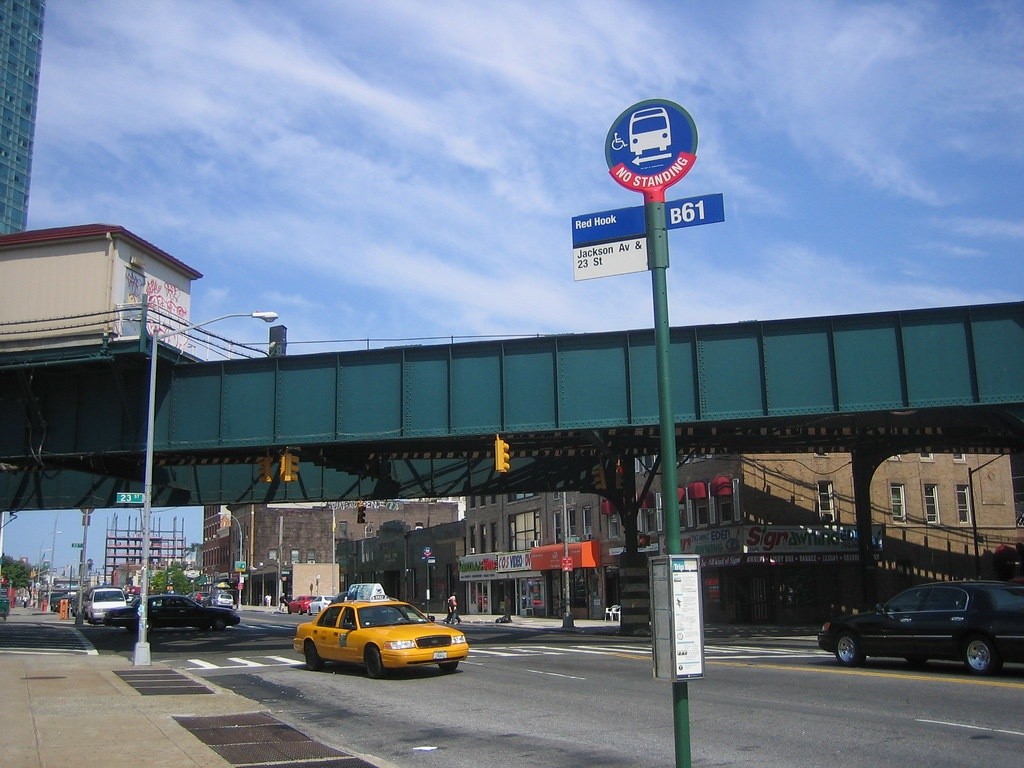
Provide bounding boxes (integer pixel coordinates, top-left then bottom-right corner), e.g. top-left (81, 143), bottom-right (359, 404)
top-left (259, 456), bottom-right (271, 483)
top-left (356, 505), bottom-right (366, 524)
top-left (495, 440), bottom-right (511, 472)
top-left (284, 454), bottom-right (299, 484)
top-left (46, 568), bottom-right (57, 572)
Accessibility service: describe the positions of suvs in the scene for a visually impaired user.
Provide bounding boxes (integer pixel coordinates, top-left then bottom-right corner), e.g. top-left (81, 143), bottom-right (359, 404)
top-left (211, 593), bottom-right (233, 609)
top-left (191, 592), bottom-right (210, 606)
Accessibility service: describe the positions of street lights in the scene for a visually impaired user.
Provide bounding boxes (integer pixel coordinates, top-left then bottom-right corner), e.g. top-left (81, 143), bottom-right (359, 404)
top-left (36, 531), bottom-right (62, 608)
top-left (134, 312), bottom-right (279, 664)
top-left (217, 512), bottom-right (242, 608)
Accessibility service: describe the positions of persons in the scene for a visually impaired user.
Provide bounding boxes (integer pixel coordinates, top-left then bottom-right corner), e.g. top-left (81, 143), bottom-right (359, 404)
top-left (280, 592), bottom-right (292, 612)
top-left (265, 593), bottom-right (272, 608)
top-left (196, 593), bottom-right (201, 605)
top-left (24, 596), bottom-right (27, 608)
top-left (443, 592), bottom-right (462, 625)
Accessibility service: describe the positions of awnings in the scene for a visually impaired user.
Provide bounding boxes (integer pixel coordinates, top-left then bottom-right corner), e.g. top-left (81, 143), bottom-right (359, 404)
top-left (710, 475), bottom-right (732, 497)
top-left (678, 487), bottom-right (685, 502)
top-left (530, 540), bottom-right (601, 571)
top-left (688, 481), bottom-right (707, 498)
top-left (601, 490), bottom-right (654, 515)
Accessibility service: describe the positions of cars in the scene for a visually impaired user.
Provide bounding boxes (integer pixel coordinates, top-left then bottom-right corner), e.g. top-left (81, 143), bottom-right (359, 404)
top-left (329, 591), bottom-right (348, 619)
top-left (292, 580), bottom-right (469, 679)
top-left (126, 593), bottom-right (134, 602)
top-left (101, 593), bottom-right (240, 632)
top-left (39, 586), bottom-right (111, 616)
top-left (288, 595), bottom-right (317, 615)
top-left (186, 592), bottom-right (193, 600)
top-left (817, 580), bottom-right (1024, 677)
top-left (307, 595), bottom-right (335, 616)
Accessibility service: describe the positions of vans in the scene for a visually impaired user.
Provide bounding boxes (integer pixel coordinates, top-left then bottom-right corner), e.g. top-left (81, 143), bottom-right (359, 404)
top-left (87, 588), bottom-right (128, 624)
